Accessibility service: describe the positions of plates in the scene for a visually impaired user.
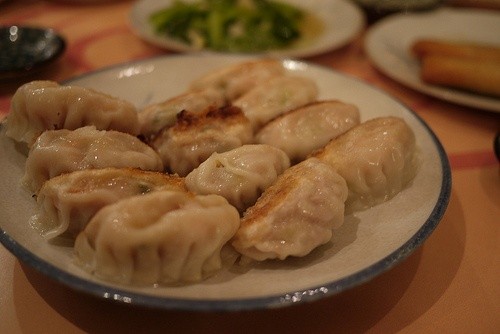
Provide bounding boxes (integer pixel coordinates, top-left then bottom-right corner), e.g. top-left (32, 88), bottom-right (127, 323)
top-left (122, 0), bottom-right (367, 58)
top-left (364, 8), bottom-right (500, 110)
top-left (0, 52), bottom-right (452, 309)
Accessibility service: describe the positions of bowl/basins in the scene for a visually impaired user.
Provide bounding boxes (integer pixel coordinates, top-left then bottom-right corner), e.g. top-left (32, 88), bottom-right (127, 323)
top-left (0, 25), bottom-right (67, 71)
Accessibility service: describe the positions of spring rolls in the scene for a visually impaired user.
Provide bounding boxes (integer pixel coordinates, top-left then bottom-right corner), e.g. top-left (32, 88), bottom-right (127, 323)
top-left (410, 36), bottom-right (500, 100)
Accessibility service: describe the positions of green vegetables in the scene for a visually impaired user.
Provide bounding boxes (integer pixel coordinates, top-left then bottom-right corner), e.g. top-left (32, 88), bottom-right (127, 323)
top-left (148, 0), bottom-right (305, 52)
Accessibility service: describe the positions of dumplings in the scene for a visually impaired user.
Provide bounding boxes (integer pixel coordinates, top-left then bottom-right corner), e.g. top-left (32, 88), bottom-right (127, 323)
top-left (0, 57), bottom-right (419, 288)
